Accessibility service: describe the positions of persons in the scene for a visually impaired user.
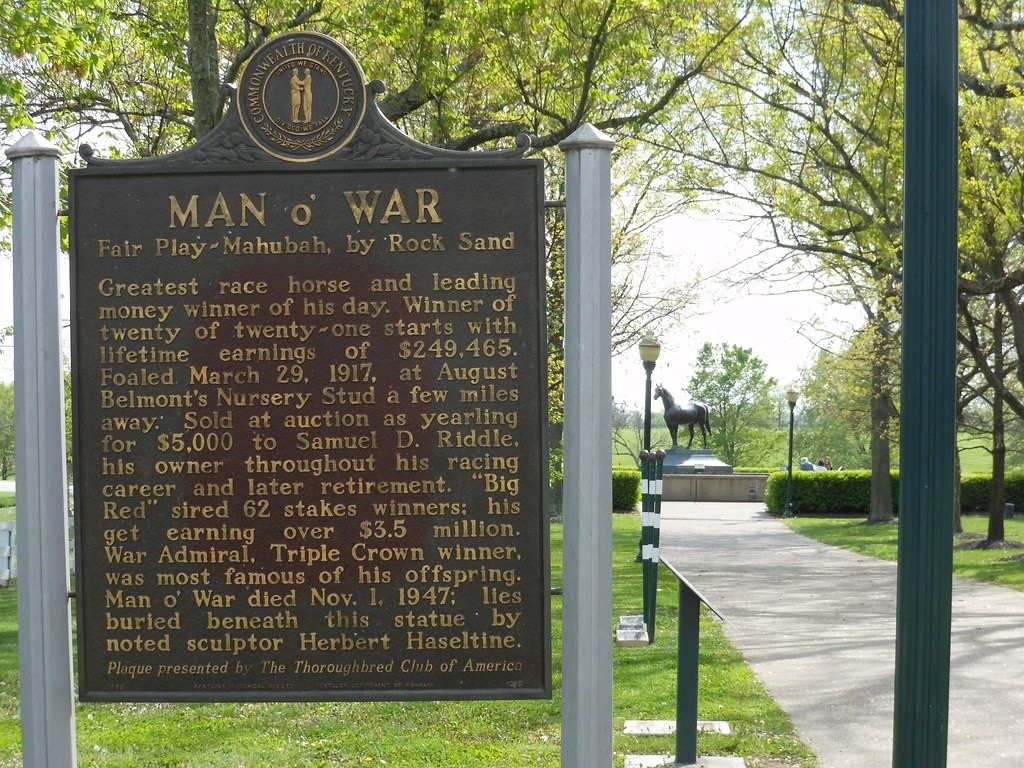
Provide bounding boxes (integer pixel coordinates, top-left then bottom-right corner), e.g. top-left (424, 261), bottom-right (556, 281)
top-left (800, 456), bottom-right (845, 471)
top-left (290, 68), bottom-right (312, 123)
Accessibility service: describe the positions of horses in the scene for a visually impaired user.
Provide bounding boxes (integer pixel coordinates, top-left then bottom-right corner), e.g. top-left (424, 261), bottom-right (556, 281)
top-left (653, 382), bottom-right (712, 450)
